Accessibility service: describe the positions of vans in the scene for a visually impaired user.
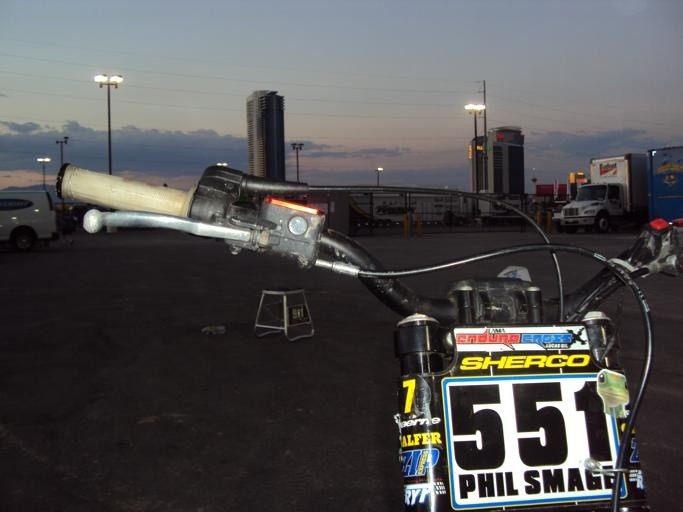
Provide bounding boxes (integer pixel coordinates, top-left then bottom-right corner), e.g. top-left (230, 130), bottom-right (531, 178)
top-left (1, 188), bottom-right (57, 252)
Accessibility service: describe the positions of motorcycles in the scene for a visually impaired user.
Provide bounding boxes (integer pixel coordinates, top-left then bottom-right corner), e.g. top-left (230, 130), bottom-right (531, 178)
top-left (49, 161), bottom-right (683, 512)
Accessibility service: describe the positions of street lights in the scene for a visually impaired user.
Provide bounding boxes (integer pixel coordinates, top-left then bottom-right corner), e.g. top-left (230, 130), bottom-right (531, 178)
top-left (375, 166), bottom-right (384, 184)
top-left (461, 102), bottom-right (488, 219)
top-left (36, 157), bottom-right (51, 187)
top-left (215, 162), bottom-right (229, 167)
top-left (95, 74), bottom-right (124, 174)
top-left (292, 142), bottom-right (303, 182)
top-left (55, 140), bottom-right (68, 167)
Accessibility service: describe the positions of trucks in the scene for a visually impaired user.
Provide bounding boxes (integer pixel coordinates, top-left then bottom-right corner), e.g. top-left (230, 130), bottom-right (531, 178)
top-left (348, 194), bottom-right (460, 229)
top-left (551, 152), bottom-right (650, 234)
